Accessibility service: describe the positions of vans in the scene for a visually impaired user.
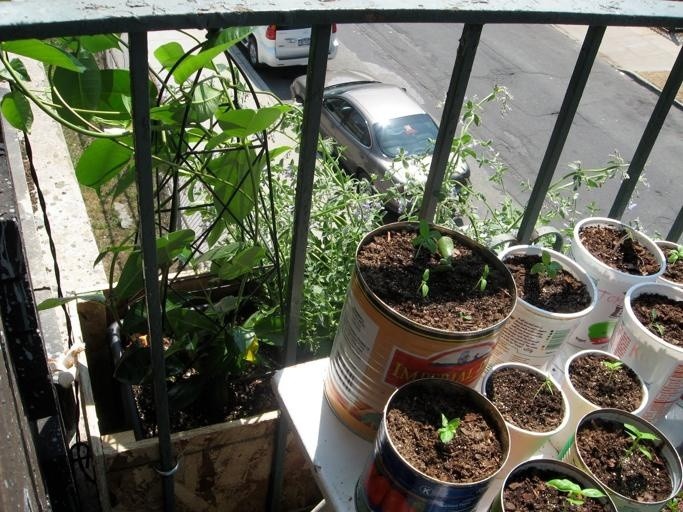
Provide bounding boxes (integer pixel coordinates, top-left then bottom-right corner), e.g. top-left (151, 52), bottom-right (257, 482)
top-left (233, 23), bottom-right (347, 67)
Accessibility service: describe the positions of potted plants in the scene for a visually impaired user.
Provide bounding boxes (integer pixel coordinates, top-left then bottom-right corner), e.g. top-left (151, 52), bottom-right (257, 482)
top-left (607, 281), bottom-right (683, 426)
top-left (652, 240), bottom-right (683, 287)
top-left (481, 361), bottom-right (570, 480)
top-left (563, 217), bottom-right (666, 349)
top-left (480, 244), bottom-right (597, 378)
top-left (549, 349), bottom-right (649, 453)
top-left (486, 459), bottom-right (618, 512)
top-left (354, 378), bottom-right (511, 512)
top-left (557, 409), bottom-right (682, 512)
top-left (324, 222), bottom-right (518, 443)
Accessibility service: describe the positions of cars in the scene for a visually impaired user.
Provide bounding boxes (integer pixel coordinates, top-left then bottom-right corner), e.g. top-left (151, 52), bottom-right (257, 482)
top-left (286, 67), bottom-right (472, 215)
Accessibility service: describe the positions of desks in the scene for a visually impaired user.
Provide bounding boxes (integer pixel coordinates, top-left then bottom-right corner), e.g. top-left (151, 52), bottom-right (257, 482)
top-left (266, 357), bottom-right (683, 512)
